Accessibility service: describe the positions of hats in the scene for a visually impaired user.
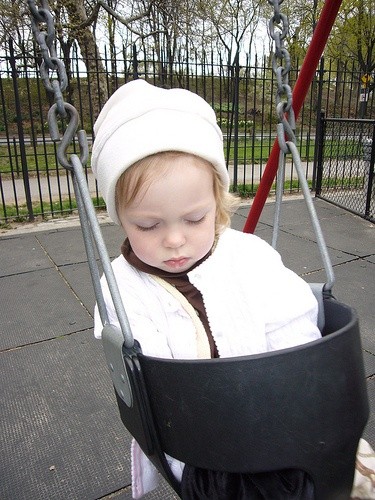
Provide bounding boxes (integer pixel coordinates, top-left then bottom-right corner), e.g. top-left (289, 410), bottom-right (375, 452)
top-left (91, 78), bottom-right (230, 225)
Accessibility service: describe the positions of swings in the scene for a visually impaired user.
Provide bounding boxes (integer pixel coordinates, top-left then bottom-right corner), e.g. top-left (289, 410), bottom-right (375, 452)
top-left (26, 0), bottom-right (372, 497)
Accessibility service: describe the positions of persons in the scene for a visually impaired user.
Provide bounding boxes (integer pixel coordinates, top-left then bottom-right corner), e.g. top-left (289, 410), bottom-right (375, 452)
top-left (89, 78), bottom-right (375, 500)
